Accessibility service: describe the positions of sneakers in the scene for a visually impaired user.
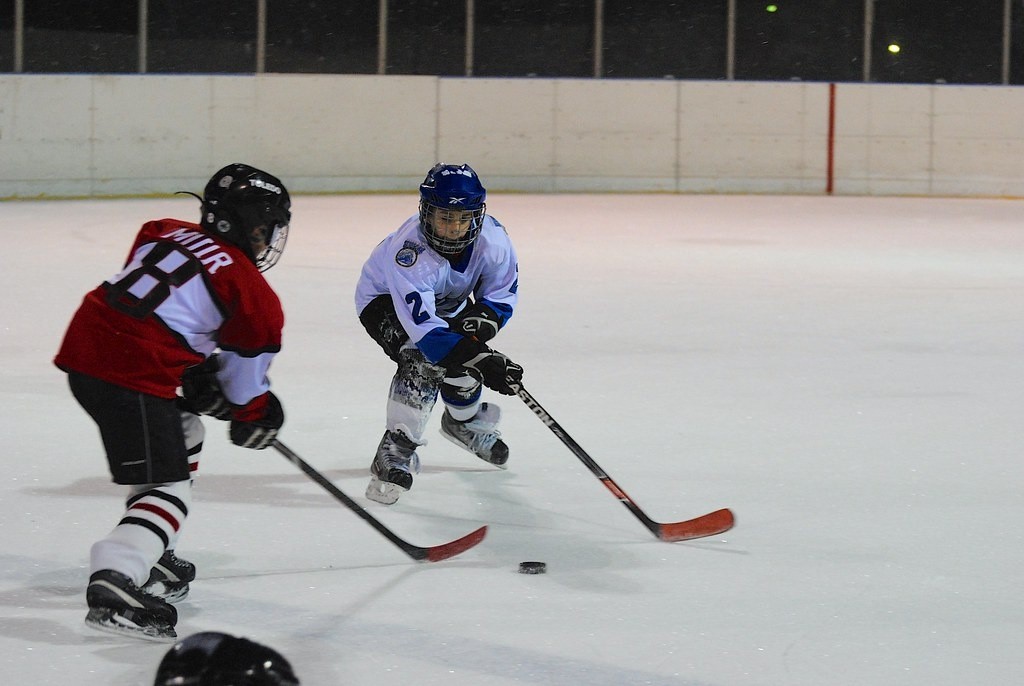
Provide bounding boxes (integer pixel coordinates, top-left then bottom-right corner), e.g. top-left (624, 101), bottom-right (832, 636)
top-left (369, 427), bottom-right (417, 490)
top-left (442, 411), bottom-right (510, 464)
top-left (87, 544), bottom-right (179, 630)
top-left (145, 550), bottom-right (196, 599)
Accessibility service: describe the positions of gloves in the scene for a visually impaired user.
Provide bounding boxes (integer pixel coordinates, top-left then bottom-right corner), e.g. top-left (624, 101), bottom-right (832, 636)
top-left (183, 354), bottom-right (228, 421)
top-left (462, 346), bottom-right (523, 396)
top-left (455, 302), bottom-right (501, 343)
top-left (229, 392), bottom-right (282, 449)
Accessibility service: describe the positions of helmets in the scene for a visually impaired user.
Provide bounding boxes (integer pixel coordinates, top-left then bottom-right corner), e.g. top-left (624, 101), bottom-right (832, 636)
top-left (419, 164), bottom-right (486, 251)
top-left (201, 162), bottom-right (291, 242)
top-left (154, 632), bottom-right (300, 686)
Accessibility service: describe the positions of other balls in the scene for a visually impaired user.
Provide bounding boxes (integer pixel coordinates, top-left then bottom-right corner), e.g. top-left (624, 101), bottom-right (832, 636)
top-left (518, 562), bottom-right (546, 575)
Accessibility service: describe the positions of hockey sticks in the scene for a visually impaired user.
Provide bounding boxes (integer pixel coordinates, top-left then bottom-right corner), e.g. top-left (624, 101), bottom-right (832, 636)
top-left (471, 333), bottom-right (735, 543)
top-left (271, 437), bottom-right (488, 563)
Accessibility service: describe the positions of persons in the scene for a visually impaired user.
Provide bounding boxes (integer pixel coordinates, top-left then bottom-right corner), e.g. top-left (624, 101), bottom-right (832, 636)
top-left (53, 162), bottom-right (292, 643)
top-left (355, 163), bottom-right (523, 505)
